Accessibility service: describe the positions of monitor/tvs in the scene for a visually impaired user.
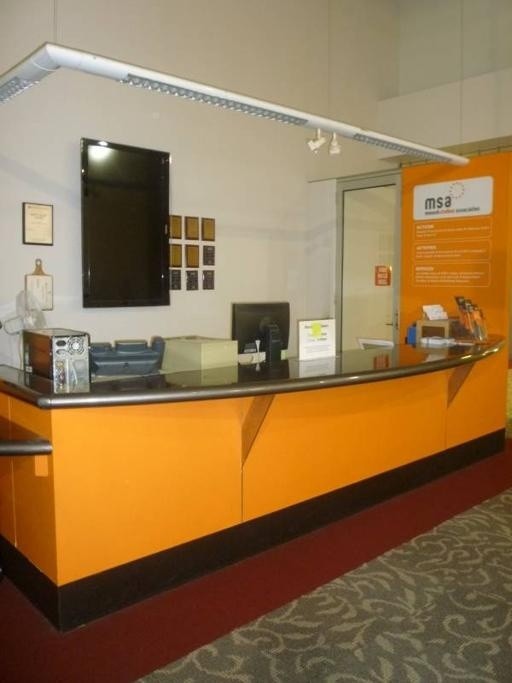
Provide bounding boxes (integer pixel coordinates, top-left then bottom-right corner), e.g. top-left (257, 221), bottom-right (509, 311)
top-left (231, 301), bottom-right (290, 363)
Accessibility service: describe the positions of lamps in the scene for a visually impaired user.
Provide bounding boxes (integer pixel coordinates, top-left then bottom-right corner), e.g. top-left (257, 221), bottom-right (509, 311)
top-left (0, 0), bottom-right (469, 166)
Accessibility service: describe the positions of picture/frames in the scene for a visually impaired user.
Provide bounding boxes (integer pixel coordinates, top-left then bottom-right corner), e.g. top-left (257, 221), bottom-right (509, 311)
top-left (169, 213), bottom-right (215, 290)
top-left (21, 202), bottom-right (53, 245)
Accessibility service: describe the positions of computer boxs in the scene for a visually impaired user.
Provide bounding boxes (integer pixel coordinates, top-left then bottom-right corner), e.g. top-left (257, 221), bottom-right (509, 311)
top-left (25, 328), bottom-right (90, 385)
